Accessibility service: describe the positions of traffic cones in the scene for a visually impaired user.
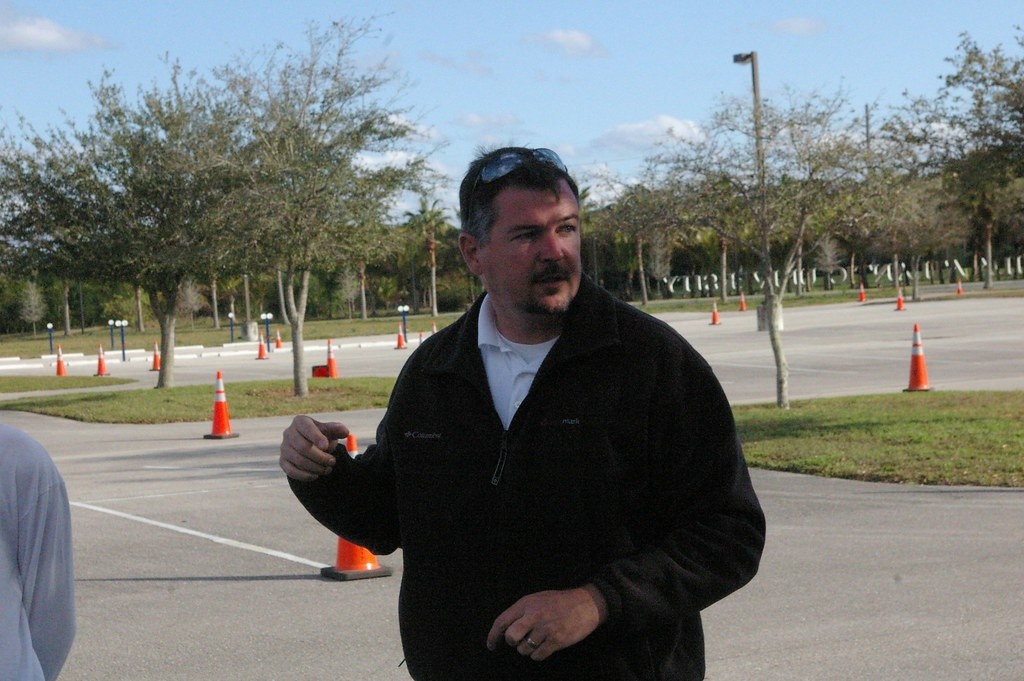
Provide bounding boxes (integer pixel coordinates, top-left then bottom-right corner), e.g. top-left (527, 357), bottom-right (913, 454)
top-left (902, 323), bottom-right (934, 392)
top-left (275, 328), bottom-right (283, 349)
top-left (148, 340), bottom-right (160, 371)
top-left (858, 280), bottom-right (866, 302)
top-left (326, 338), bottom-right (338, 378)
top-left (56, 343), bottom-right (67, 376)
top-left (738, 291), bottom-right (747, 312)
top-left (955, 278), bottom-right (964, 295)
top-left (93, 344), bottom-right (111, 377)
top-left (254, 330), bottom-right (270, 360)
top-left (708, 299), bottom-right (722, 326)
top-left (203, 371), bottom-right (240, 439)
top-left (893, 288), bottom-right (907, 312)
top-left (394, 323), bottom-right (407, 350)
top-left (431, 322), bottom-right (437, 335)
top-left (318, 432), bottom-right (394, 582)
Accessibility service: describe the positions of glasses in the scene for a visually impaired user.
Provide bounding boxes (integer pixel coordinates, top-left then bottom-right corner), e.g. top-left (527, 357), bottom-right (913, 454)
top-left (466, 148), bottom-right (568, 218)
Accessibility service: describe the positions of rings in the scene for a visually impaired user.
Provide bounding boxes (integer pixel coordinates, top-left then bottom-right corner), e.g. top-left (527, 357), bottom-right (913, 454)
top-left (526, 636), bottom-right (538, 649)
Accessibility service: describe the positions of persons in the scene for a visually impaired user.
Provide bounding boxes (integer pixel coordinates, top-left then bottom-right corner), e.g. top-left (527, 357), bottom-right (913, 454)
top-left (279, 147), bottom-right (766, 681)
top-left (0, 422), bottom-right (77, 681)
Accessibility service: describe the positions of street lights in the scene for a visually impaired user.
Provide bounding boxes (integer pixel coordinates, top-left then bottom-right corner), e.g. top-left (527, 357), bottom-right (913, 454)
top-left (731, 49), bottom-right (777, 305)
